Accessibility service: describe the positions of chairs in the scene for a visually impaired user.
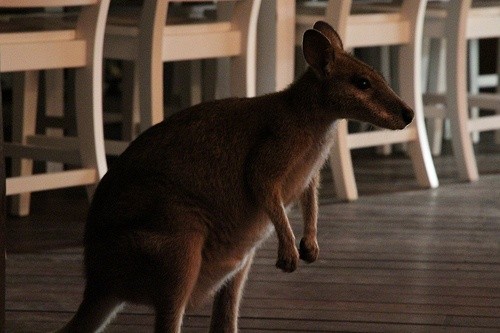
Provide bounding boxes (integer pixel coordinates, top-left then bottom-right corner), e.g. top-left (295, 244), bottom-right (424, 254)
top-left (0, 0), bottom-right (110, 216)
top-left (42, 0), bottom-right (261, 173)
top-left (257, 0), bottom-right (439, 200)
top-left (422, 0), bottom-right (500, 180)
